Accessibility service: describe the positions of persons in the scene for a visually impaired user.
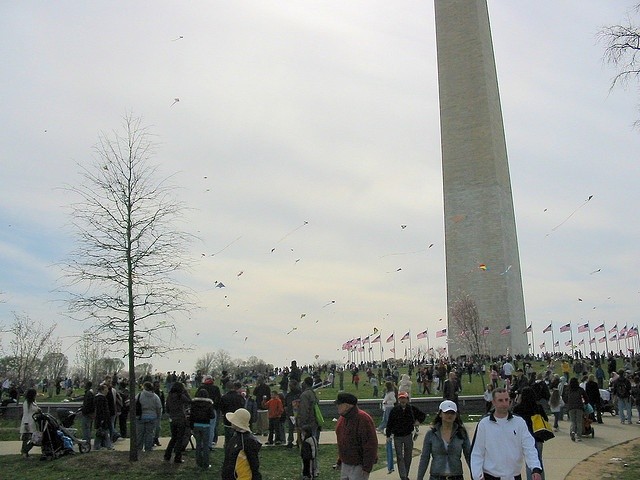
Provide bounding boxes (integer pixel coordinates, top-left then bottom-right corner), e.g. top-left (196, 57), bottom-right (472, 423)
top-left (303, 361), bottom-right (437, 393)
top-left (276, 392), bottom-right (288, 445)
top-left (547, 389), bottom-right (565, 431)
top-left (609, 372), bottom-right (619, 414)
top-left (513, 388), bottom-right (549, 480)
top-left (387, 393), bottom-right (426, 480)
top-left (125, 382), bottom-right (162, 450)
top-left (470, 387), bottom-right (543, 480)
top-left (80, 381), bottom-right (93, 447)
top-left (625, 371), bottom-right (635, 388)
top-left (244, 386), bottom-right (257, 436)
top-left (152, 380), bottom-right (164, 447)
top-left (20, 390), bottom-right (41, 458)
top-left (333, 392), bottom-right (378, 480)
top-left (95, 385), bottom-right (113, 449)
top-left (296, 377), bottom-right (321, 446)
top-left (299, 424), bottom-right (317, 480)
top-left (223, 406), bottom-right (262, 480)
top-left (378, 381), bottom-right (397, 430)
top-left (220, 382), bottom-right (245, 449)
top-left (615, 370), bottom-right (632, 424)
top-left (134, 369), bottom-right (190, 383)
top-left (163, 382), bottom-right (191, 463)
top-left (100, 376), bottom-right (119, 441)
top-left (194, 374), bottom-right (221, 449)
top-left (117, 380), bottom-right (129, 440)
top-left (586, 374), bottom-right (604, 424)
top-left (632, 375), bottom-right (640, 425)
top-left (568, 377), bottom-right (588, 442)
top-left (380, 379), bottom-right (397, 474)
top-left (439, 354), bottom-right (640, 388)
top-left (222, 360), bottom-right (300, 381)
top-left (417, 400), bottom-right (474, 480)
top-left (285, 381), bottom-right (301, 446)
top-left (443, 371), bottom-right (461, 424)
top-left (262, 391), bottom-right (283, 446)
top-left (190, 389), bottom-right (215, 468)
top-left (254, 376), bottom-right (270, 436)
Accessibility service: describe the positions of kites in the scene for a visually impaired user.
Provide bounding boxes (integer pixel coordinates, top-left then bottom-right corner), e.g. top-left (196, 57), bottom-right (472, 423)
top-left (589, 268), bottom-right (602, 276)
top-left (236, 270), bottom-right (245, 278)
top-left (323, 300), bottom-right (336, 308)
top-left (577, 297), bottom-right (583, 302)
top-left (243, 336), bottom-right (248, 342)
top-left (294, 257), bottom-right (302, 264)
top-left (269, 247), bottom-right (276, 254)
top-left (286, 327), bottom-right (298, 336)
top-left (541, 208), bottom-right (548, 213)
top-left (203, 189), bottom-right (211, 193)
top-left (427, 243), bottom-right (434, 250)
top-left (400, 224), bottom-right (407, 231)
top-left (215, 281), bottom-right (225, 290)
top-left (584, 193), bottom-right (594, 205)
top-left (299, 313), bottom-right (307, 319)
top-left (170, 98), bottom-right (180, 107)
top-left (202, 176), bottom-right (209, 181)
top-left (314, 354), bottom-right (319, 361)
top-left (200, 252), bottom-right (206, 261)
top-left (437, 346), bottom-right (446, 361)
top-left (301, 220), bottom-right (309, 228)
top-left (170, 34), bottom-right (185, 42)
top-left (396, 267), bottom-right (402, 273)
top-left (560, 320), bottom-right (574, 335)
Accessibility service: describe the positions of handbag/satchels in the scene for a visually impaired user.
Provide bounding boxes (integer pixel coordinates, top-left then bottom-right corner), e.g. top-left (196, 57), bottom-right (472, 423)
top-left (532, 402), bottom-right (555, 443)
top-left (136, 394), bottom-right (142, 418)
top-left (315, 403), bottom-right (325, 427)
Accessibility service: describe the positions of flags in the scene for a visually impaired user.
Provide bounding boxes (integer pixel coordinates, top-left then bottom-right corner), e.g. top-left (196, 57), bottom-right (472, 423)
top-left (543, 320), bottom-right (555, 335)
top-left (363, 336), bottom-right (372, 344)
top-left (634, 325), bottom-right (640, 339)
top-left (610, 334), bottom-right (618, 344)
top-left (598, 336), bottom-right (607, 346)
top-left (578, 338), bottom-right (586, 349)
top-left (540, 340), bottom-right (547, 350)
top-left (499, 325), bottom-right (512, 339)
top-left (386, 329), bottom-right (396, 343)
top-left (435, 328), bottom-right (448, 341)
top-left (416, 327), bottom-right (430, 343)
top-left (565, 339), bottom-right (574, 348)
top-left (609, 322), bottom-right (619, 337)
top-left (481, 327), bottom-right (493, 338)
top-left (401, 329), bottom-right (411, 345)
top-left (577, 320), bottom-right (593, 334)
top-left (594, 321), bottom-right (608, 334)
top-left (528, 343), bottom-right (532, 354)
top-left (628, 323), bottom-right (635, 340)
top-left (521, 321), bottom-right (535, 336)
top-left (390, 347), bottom-right (396, 356)
top-left (620, 323), bottom-right (628, 341)
top-left (339, 336), bottom-right (363, 354)
top-left (554, 339), bottom-right (559, 349)
top-left (589, 337), bottom-right (597, 347)
top-left (0, 372), bottom-right (96, 394)
top-left (371, 333), bottom-right (382, 345)
top-left (457, 326), bottom-right (471, 342)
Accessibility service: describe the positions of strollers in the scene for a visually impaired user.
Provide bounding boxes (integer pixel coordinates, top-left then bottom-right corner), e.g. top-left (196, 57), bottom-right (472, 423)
top-left (32, 410), bottom-right (91, 461)
top-left (570, 404), bottom-right (594, 438)
top-left (58, 408), bottom-right (82, 426)
top-left (595, 398), bottom-right (617, 416)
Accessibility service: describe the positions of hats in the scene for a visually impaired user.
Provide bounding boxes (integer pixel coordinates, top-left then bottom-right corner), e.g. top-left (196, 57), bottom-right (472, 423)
top-left (334, 392), bottom-right (357, 405)
top-left (439, 400), bottom-right (457, 412)
top-left (398, 391), bottom-right (408, 398)
top-left (226, 408), bottom-right (252, 433)
